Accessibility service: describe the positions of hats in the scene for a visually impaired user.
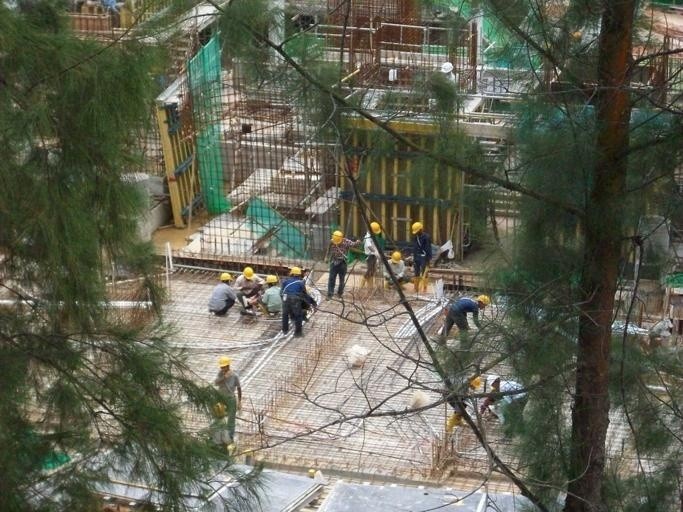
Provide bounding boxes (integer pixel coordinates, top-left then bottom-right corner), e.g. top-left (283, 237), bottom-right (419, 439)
top-left (476, 294), bottom-right (490, 305)
top-left (332, 230), bottom-right (343, 245)
top-left (368, 221), bottom-right (381, 235)
top-left (217, 356), bottom-right (231, 368)
top-left (486, 374), bottom-right (501, 387)
top-left (411, 221), bottom-right (424, 235)
top-left (468, 375), bottom-right (481, 387)
top-left (219, 272), bottom-right (234, 282)
top-left (391, 250), bottom-right (402, 264)
top-left (264, 275), bottom-right (279, 285)
top-left (243, 267), bottom-right (255, 280)
top-left (289, 266), bottom-right (302, 276)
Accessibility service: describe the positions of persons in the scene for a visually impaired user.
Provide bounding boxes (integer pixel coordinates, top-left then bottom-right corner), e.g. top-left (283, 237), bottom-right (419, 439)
top-left (440, 61), bottom-right (456, 85)
top-left (69, 0), bottom-right (116, 14)
top-left (166, 91), bottom-right (182, 107)
top-left (214, 357), bottom-right (242, 438)
top-left (439, 294), bottom-right (490, 348)
top-left (443, 373), bottom-right (530, 444)
top-left (325, 231), bottom-right (360, 300)
top-left (207, 266), bottom-right (318, 338)
top-left (411, 222), bottom-right (434, 294)
top-left (359, 222), bottom-right (381, 292)
top-left (208, 402), bottom-right (233, 460)
top-left (382, 251), bottom-right (412, 292)
